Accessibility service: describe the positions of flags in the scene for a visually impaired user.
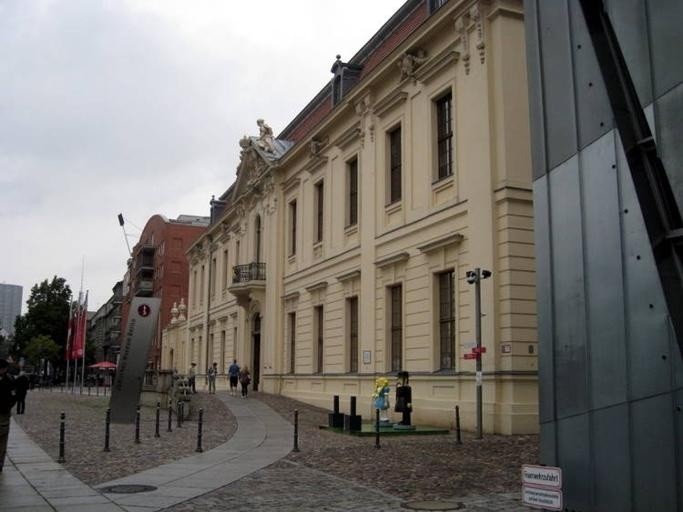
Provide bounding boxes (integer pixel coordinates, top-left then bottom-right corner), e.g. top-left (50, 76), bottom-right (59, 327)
top-left (64, 305), bottom-right (87, 360)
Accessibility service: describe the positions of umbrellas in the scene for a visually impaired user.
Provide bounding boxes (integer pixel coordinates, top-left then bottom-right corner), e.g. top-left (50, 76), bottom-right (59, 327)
top-left (88, 361), bottom-right (116, 384)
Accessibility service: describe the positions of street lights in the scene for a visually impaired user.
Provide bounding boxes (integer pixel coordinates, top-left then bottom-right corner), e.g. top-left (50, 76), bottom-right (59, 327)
top-left (465, 267), bottom-right (492, 439)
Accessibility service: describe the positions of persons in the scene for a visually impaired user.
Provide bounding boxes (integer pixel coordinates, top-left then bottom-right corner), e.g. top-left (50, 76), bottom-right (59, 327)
top-left (208, 362), bottom-right (218, 394)
top-left (257, 119), bottom-right (277, 155)
top-left (239, 365), bottom-right (250, 398)
top-left (394, 371), bottom-right (413, 425)
top-left (0, 358), bottom-right (30, 473)
top-left (187, 363), bottom-right (197, 393)
top-left (373, 377), bottom-right (390, 422)
top-left (227, 360), bottom-right (241, 397)
